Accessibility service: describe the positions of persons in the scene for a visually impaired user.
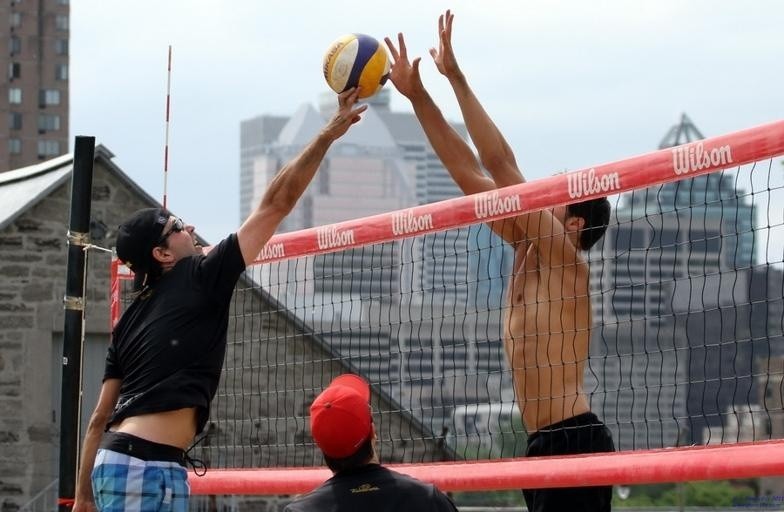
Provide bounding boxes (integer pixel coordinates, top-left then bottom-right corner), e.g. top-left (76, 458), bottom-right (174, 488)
top-left (72, 87), bottom-right (368, 512)
top-left (385, 9), bottom-right (615, 512)
top-left (282, 374), bottom-right (457, 512)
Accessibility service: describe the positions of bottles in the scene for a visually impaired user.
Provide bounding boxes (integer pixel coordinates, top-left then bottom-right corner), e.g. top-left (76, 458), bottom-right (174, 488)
top-left (117, 206), bottom-right (169, 292)
top-left (309, 373), bottom-right (371, 459)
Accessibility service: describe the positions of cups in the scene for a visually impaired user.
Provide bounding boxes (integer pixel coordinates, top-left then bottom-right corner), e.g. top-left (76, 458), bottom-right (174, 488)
top-left (160, 218), bottom-right (184, 245)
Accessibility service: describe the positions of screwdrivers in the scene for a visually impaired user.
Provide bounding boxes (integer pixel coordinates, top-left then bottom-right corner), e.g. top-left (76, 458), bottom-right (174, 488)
top-left (322, 33), bottom-right (390, 98)
